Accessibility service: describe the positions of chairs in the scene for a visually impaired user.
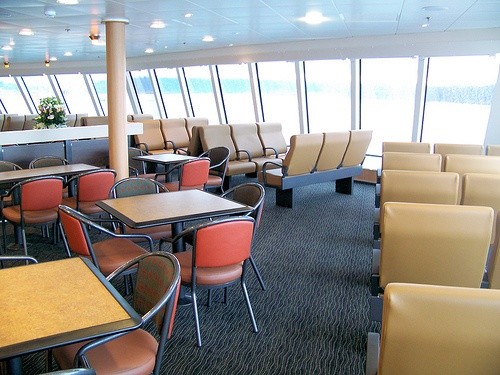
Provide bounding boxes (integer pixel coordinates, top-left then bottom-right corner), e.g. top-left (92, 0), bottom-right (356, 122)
top-left (0, 114), bottom-right (500, 375)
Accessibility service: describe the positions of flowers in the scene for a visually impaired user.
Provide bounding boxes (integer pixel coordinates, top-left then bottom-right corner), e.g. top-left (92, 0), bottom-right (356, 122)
top-left (33, 97), bottom-right (67, 129)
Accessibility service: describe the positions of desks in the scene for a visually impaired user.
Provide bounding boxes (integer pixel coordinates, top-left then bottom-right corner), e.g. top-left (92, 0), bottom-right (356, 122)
top-left (96, 189), bottom-right (252, 306)
top-left (0, 163), bottom-right (100, 249)
top-left (135, 154), bottom-right (198, 181)
top-left (0, 255), bottom-right (142, 375)
